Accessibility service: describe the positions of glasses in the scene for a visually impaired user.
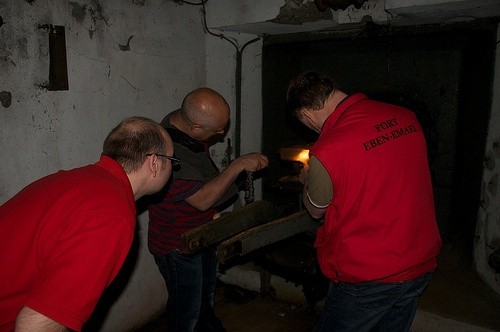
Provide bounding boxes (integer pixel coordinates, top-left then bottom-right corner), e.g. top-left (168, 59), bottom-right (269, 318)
top-left (156, 153), bottom-right (180, 166)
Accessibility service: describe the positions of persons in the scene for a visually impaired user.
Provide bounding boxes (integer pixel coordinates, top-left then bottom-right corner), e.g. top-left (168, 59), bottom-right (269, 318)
top-left (148, 88), bottom-right (269, 332)
top-left (0, 116), bottom-right (173, 332)
top-left (287, 71), bottom-right (443, 332)
